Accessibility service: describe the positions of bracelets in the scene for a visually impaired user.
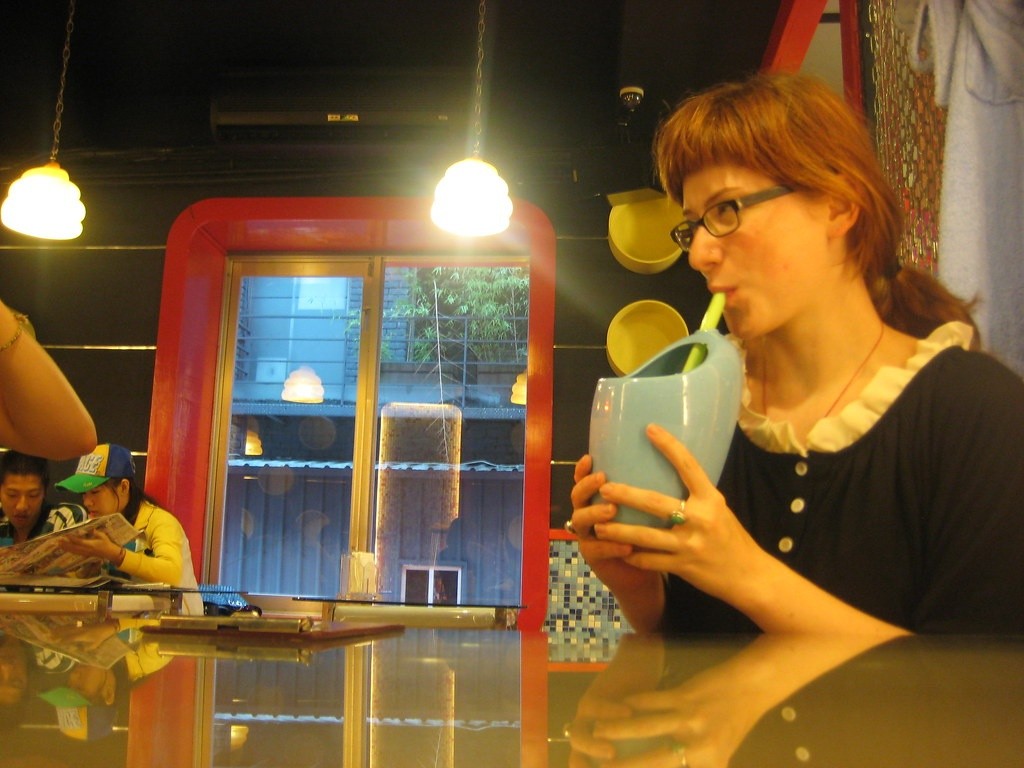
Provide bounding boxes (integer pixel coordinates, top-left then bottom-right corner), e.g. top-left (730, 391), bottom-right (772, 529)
top-left (0, 315), bottom-right (26, 352)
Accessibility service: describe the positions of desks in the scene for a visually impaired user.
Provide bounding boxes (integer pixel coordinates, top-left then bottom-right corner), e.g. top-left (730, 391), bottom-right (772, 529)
top-left (0, 584), bottom-right (250, 612)
top-left (291, 595), bottom-right (527, 631)
top-left (0, 611), bottom-right (1024, 768)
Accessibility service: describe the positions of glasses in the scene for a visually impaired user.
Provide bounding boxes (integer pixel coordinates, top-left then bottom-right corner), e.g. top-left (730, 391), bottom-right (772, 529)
top-left (670, 182), bottom-right (798, 250)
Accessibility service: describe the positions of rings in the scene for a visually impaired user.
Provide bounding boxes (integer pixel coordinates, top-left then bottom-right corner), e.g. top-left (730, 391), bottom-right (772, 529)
top-left (670, 500), bottom-right (687, 525)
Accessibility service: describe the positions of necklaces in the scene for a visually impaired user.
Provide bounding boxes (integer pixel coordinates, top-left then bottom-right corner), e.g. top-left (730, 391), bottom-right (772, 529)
top-left (762, 322), bottom-right (886, 435)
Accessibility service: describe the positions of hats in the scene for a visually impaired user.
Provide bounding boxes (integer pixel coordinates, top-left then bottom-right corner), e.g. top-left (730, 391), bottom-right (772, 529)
top-left (55, 443), bottom-right (137, 494)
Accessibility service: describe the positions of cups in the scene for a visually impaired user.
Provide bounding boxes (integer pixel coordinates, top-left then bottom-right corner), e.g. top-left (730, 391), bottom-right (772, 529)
top-left (588, 332), bottom-right (742, 526)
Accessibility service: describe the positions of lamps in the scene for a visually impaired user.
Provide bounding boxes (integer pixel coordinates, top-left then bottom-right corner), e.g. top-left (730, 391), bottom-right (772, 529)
top-left (0, 0), bottom-right (86, 240)
top-left (430, 0), bottom-right (512, 238)
top-left (510, 369), bottom-right (527, 405)
top-left (281, 366), bottom-right (324, 404)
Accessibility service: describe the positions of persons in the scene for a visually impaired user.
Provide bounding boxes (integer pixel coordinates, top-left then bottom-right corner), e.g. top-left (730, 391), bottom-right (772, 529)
top-left (571, 73), bottom-right (1024, 637)
top-left (0, 298), bottom-right (205, 621)
